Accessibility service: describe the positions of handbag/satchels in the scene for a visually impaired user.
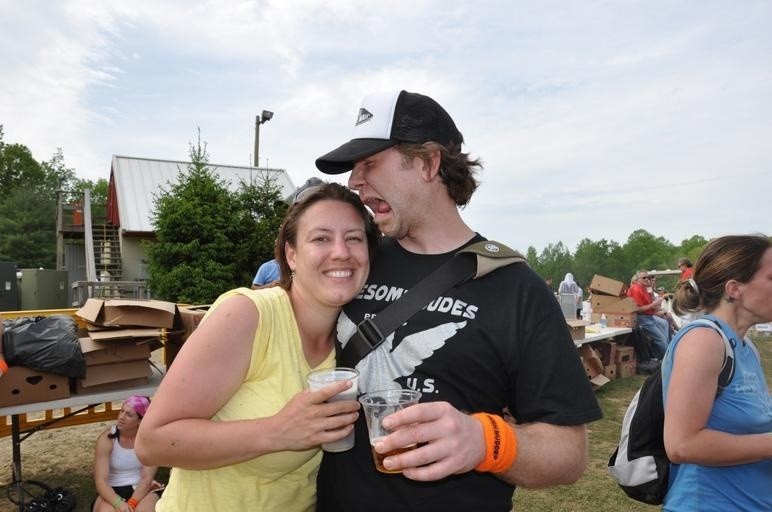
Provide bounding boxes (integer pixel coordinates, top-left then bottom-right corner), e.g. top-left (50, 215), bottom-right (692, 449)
top-left (7, 479), bottom-right (77, 512)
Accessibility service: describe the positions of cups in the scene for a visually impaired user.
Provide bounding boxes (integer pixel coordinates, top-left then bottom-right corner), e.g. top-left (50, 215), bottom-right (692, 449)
top-left (359, 389), bottom-right (423, 474)
top-left (306, 367), bottom-right (360, 453)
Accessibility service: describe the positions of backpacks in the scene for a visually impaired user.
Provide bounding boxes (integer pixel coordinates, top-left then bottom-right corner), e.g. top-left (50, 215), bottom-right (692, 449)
top-left (607, 319), bottom-right (736, 506)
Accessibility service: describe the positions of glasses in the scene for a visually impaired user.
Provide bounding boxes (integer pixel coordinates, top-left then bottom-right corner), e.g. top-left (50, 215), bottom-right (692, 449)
top-left (292, 185), bottom-right (323, 207)
top-left (640, 276), bottom-right (649, 279)
top-left (678, 264), bottom-right (682, 268)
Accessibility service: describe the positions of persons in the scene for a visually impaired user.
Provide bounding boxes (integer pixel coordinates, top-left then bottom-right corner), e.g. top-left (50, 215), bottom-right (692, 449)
top-left (618, 258), bottom-right (693, 369)
top-left (607, 234), bottom-right (772, 511)
top-left (542, 268), bottom-right (584, 319)
top-left (251, 239), bottom-right (282, 290)
top-left (133, 182), bottom-right (369, 512)
top-left (89, 396), bottom-right (162, 512)
top-left (317, 85), bottom-right (602, 511)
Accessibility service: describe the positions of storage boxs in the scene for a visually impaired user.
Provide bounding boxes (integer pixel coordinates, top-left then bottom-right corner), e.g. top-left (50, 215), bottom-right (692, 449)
top-left (0, 294), bottom-right (216, 411)
top-left (589, 275), bottom-right (638, 329)
top-left (566, 317), bottom-right (587, 340)
top-left (578, 338), bottom-right (636, 391)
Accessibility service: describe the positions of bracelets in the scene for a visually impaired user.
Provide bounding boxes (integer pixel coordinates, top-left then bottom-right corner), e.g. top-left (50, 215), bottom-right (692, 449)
top-left (127, 497), bottom-right (138, 508)
top-left (112, 496), bottom-right (123, 509)
top-left (472, 410), bottom-right (517, 475)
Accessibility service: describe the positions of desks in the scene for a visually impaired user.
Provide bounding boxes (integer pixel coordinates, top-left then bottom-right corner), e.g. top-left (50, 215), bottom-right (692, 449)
top-left (571, 327), bottom-right (634, 355)
top-left (2, 358), bottom-right (184, 512)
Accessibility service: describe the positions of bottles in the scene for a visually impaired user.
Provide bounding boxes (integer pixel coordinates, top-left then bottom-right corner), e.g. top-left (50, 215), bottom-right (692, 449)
top-left (600, 314), bottom-right (607, 330)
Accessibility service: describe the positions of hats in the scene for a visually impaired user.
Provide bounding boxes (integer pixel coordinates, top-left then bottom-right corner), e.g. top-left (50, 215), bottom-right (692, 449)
top-left (315, 89), bottom-right (462, 175)
